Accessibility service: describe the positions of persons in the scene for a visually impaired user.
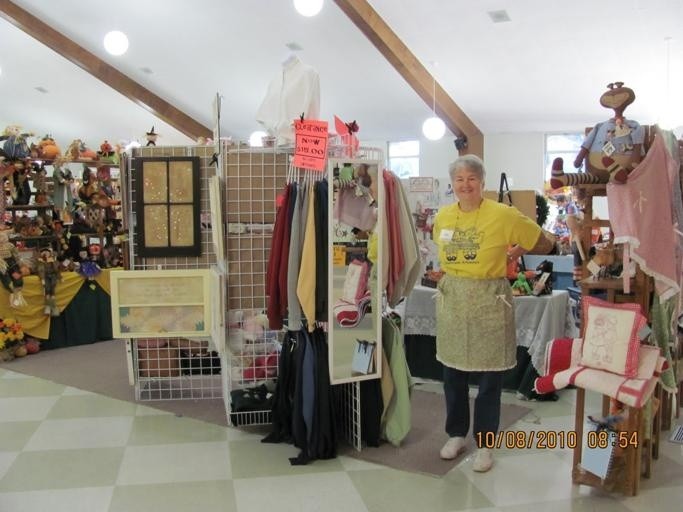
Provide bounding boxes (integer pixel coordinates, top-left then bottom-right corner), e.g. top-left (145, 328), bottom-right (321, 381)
top-left (557, 196), bottom-right (578, 228)
top-left (431, 154), bottom-right (554, 471)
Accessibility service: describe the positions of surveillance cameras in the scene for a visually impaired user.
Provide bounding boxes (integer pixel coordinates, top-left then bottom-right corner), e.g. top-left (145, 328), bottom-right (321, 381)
top-left (455, 137), bottom-right (467, 151)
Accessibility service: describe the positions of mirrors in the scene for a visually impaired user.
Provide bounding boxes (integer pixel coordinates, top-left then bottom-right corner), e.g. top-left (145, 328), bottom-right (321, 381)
top-left (326, 157), bottom-right (383, 386)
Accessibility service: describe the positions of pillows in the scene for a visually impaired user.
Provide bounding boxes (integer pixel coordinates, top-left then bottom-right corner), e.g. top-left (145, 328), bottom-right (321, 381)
top-left (576, 296), bottom-right (648, 381)
top-left (339, 259), bottom-right (367, 305)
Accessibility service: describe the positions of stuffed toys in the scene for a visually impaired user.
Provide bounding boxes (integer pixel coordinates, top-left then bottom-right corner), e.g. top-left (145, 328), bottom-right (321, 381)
top-left (135, 337), bottom-right (222, 377)
top-left (226, 309), bottom-right (283, 424)
top-left (0, 124), bottom-right (283, 424)
top-left (550, 80), bottom-right (645, 189)
top-left (145, 126), bottom-right (158, 147)
top-left (1, 125), bottom-right (141, 361)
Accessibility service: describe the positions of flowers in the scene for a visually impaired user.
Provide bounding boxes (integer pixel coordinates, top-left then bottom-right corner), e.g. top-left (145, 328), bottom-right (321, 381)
top-left (1, 317), bottom-right (26, 347)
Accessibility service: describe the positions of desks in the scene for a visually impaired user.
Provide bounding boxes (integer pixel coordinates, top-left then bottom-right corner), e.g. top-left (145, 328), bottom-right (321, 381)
top-left (402, 284), bottom-right (570, 402)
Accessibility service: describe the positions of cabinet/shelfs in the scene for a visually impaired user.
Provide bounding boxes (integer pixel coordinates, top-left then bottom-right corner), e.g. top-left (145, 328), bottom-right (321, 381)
top-left (1, 153), bottom-right (128, 347)
top-left (566, 181), bottom-right (683, 498)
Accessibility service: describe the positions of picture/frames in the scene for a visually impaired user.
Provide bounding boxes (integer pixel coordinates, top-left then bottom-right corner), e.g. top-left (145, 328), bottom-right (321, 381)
top-left (106, 268), bottom-right (213, 341)
top-left (135, 155), bottom-right (201, 259)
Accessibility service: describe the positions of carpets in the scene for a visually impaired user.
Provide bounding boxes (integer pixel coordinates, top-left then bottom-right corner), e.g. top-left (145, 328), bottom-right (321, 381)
top-left (0, 340), bottom-right (534, 479)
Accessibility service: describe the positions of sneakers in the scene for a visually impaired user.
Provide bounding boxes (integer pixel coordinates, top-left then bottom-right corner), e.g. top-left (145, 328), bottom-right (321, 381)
top-left (440, 436), bottom-right (464, 458)
top-left (473, 448), bottom-right (492, 471)
